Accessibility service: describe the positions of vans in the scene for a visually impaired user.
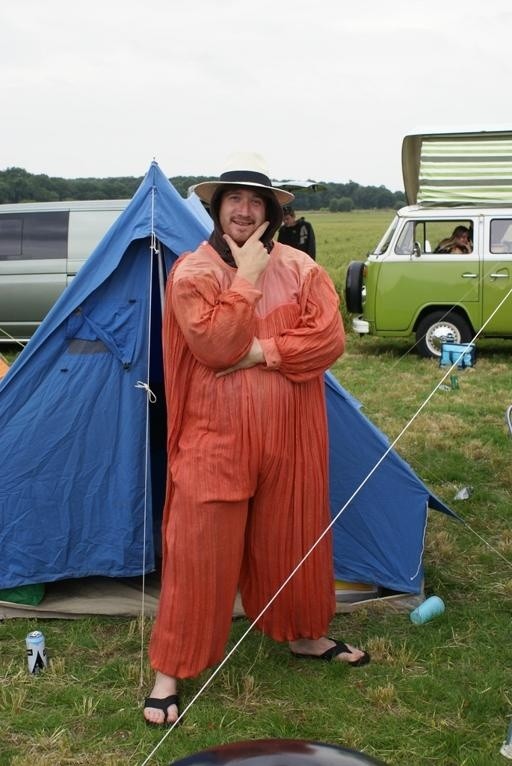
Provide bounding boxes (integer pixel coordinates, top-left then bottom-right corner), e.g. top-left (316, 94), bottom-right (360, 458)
top-left (1, 179), bottom-right (215, 353)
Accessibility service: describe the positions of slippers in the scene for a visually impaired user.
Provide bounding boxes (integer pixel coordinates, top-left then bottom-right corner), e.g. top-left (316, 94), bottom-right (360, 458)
top-left (142, 695), bottom-right (178, 728)
top-left (289, 638), bottom-right (368, 667)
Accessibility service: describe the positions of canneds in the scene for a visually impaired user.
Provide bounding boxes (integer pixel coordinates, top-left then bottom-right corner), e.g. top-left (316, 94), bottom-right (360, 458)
top-left (26, 630), bottom-right (46, 674)
top-left (451, 375), bottom-right (459, 390)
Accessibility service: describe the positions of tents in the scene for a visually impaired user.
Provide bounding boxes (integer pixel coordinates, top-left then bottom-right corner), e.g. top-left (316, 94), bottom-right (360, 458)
top-left (0, 161), bottom-right (466, 623)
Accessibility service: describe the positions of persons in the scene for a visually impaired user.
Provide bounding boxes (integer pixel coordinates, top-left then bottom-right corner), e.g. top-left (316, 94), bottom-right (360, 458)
top-left (277, 206), bottom-right (315, 261)
top-left (141, 156), bottom-right (371, 729)
top-left (434, 226), bottom-right (469, 253)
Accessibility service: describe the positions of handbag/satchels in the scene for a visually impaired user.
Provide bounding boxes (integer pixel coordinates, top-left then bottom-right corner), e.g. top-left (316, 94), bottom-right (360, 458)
top-left (438, 342), bottom-right (475, 370)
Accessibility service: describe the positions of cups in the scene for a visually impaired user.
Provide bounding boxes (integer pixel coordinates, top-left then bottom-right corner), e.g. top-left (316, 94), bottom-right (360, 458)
top-left (410, 596), bottom-right (446, 625)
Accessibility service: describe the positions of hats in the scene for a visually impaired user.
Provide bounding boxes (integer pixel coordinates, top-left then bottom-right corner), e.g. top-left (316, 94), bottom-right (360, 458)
top-left (194, 153), bottom-right (294, 205)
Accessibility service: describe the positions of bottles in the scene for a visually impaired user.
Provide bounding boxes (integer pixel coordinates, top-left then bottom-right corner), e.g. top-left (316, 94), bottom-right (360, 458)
top-left (446, 333), bottom-right (454, 344)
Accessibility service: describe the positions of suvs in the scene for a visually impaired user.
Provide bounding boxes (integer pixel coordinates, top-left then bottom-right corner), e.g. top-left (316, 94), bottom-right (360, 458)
top-left (343, 204), bottom-right (511, 361)
